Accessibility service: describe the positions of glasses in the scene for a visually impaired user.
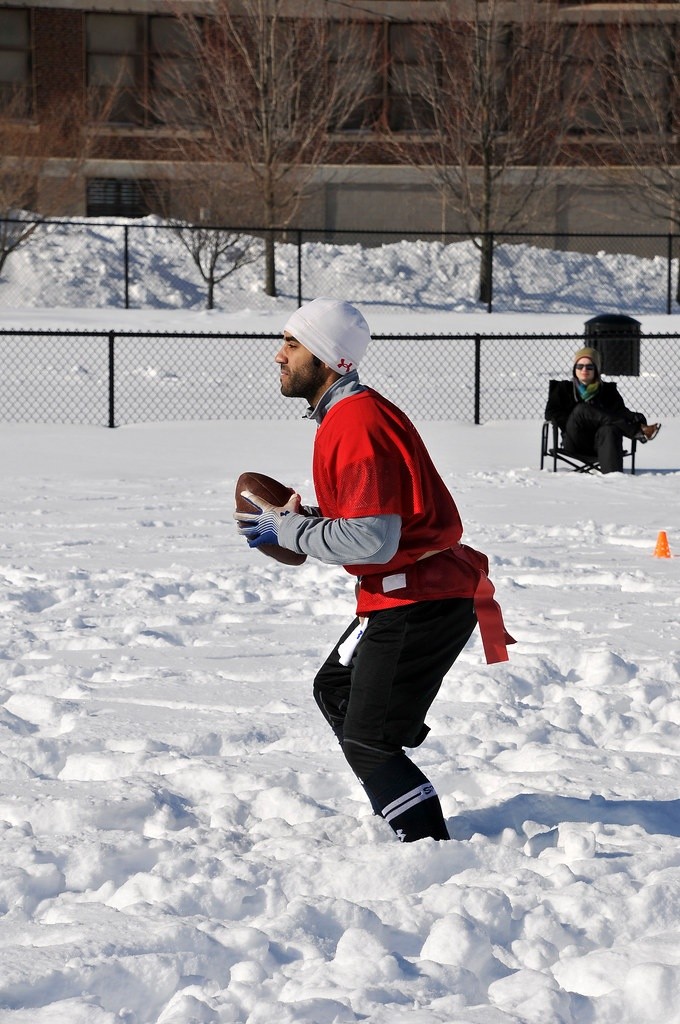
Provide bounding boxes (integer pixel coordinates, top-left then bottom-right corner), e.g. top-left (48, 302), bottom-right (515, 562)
top-left (575, 364), bottom-right (596, 371)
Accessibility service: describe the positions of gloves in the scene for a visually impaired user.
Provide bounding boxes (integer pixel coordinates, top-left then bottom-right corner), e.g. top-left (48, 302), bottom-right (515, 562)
top-left (232, 490), bottom-right (301, 548)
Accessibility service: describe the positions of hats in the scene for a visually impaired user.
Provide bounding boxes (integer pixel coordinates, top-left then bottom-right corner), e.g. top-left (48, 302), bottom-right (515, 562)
top-left (573, 347), bottom-right (600, 377)
top-left (284, 296), bottom-right (370, 375)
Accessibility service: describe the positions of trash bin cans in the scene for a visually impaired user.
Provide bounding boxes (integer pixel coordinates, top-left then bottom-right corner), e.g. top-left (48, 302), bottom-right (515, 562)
top-left (584, 315), bottom-right (641, 377)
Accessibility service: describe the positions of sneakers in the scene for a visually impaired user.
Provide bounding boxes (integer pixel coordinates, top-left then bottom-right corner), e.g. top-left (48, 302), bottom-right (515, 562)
top-left (635, 423), bottom-right (661, 444)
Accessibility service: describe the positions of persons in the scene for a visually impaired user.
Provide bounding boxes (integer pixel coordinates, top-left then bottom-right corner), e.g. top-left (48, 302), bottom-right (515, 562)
top-left (542, 347), bottom-right (662, 473)
top-left (233, 296), bottom-right (517, 844)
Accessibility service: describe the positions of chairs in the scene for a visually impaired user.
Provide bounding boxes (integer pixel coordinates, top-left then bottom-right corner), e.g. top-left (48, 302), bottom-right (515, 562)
top-left (541, 379), bottom-right (636, 475)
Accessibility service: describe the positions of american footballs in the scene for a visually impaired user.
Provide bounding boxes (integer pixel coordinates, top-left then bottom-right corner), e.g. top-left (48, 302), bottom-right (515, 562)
top-left (235, 471), bottom-right (308, 566)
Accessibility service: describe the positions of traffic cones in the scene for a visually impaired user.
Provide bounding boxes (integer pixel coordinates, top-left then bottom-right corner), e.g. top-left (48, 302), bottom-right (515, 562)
top-left (652, 531), bottom-right (673, 559)
